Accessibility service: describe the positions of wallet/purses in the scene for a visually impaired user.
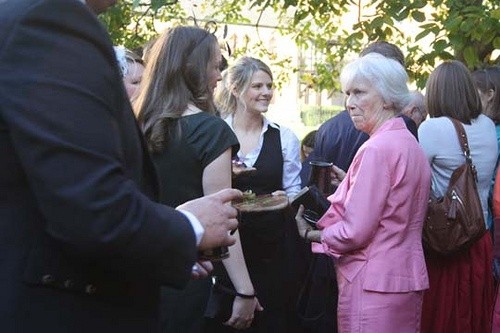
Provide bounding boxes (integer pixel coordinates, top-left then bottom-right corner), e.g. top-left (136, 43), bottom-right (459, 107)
top-left (290, 186), bottom-right (328, 228)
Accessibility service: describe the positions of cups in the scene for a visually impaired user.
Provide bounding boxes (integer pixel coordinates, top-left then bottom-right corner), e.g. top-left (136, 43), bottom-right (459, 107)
top-left (308, 161), bottom-right (333, 194)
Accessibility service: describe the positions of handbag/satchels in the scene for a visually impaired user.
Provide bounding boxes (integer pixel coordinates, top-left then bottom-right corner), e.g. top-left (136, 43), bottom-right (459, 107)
top-left (203, 282), bottom-right (235, 322)
top-left (423, 113), bottom-right (487, 256)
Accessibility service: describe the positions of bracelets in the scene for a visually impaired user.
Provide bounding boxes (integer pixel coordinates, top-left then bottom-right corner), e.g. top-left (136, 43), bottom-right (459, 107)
top-left (304, 229), bottom-right (310, 243)
top-left (235, 291), bottom-right (256, 299)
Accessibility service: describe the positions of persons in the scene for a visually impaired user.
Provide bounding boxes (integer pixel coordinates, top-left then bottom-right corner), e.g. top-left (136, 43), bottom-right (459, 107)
top-left (298, 40), bottom-right (500, 333)
top-left (210, 52), bottom-right (313, 333)
top-left (108, 40), bottom-right (148, 104)
top-left (125, 25), bottom-right (266, 333)
top-left (0, 0), bottom-right (245, 333)
